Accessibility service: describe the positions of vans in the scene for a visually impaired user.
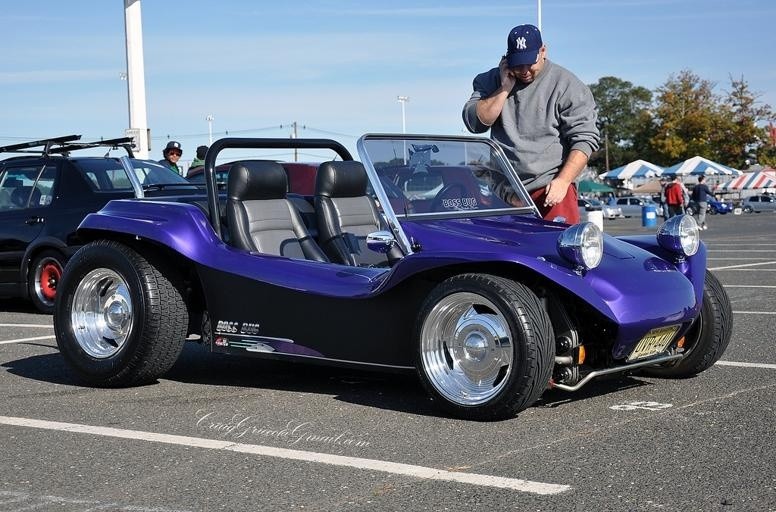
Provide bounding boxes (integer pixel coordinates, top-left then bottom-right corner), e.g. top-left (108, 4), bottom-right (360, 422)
top-left (685, 189), bottom-right (735, 215)
top-left (740, 190), bottom-right (776, 216)
top-left (383, 164), bottom-right (491, 214)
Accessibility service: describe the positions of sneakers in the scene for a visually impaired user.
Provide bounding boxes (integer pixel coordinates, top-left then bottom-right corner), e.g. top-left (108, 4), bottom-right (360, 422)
top-left (697, 225), bottom-right (703, 231)
top-left (619, 214), bottom-right (626, 218)
top-left (703, 225), bottom-right (708, 231)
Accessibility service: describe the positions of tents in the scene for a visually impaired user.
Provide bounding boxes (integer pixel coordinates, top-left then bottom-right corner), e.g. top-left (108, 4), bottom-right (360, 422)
top-left (711, 171), bottom-right (776, 197)
top-left (578, 179), bottom-right (617, 198)
top-left (630, 180), bottom-right (662, 197)
top-left (654, 156), bottom-right (743, 201)
top-left (595, 159), bottom-right (665, 197)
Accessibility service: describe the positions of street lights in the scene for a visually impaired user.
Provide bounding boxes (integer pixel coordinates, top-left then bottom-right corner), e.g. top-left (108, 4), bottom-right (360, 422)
top-left (397, 95), bottom-right (411, 192)
top-left (462, 128), bottom-right (471, 165)
top-left (207, 113), bottom-right (213, 147)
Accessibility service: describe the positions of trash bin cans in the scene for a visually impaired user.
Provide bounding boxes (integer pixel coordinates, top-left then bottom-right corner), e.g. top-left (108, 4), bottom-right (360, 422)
top-left (642, 205), bottom-right (657, 228)
top-left (585, 205), bottom-right (602, 232)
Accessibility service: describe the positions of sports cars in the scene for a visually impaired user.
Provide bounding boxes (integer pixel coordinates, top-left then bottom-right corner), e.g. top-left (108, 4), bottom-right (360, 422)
top-left (42, 131), bottom-right (732, 421)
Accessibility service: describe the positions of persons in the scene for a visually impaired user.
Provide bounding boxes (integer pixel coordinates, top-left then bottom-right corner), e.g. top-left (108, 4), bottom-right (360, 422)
top-left (186, 145), bottom-right (209, 178)
top-left (605, 192), bottom-right (617, 206)
top-left (658, 178), bottom-right (670, 221)
top-left (142, 141), bottom-right (183, 188)
top-left (692, 175), bottom-right (716, 231)
top-left (676, 176), bottom-right (689, 214)
top-left (462, 25), bottom-right (601, 224)
top-left (664, 173), bottom-right (684, 219)
top-left (579, 191), bottom-right (602, 202)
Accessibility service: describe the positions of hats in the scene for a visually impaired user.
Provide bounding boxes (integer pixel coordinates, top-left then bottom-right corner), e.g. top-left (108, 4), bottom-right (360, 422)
top-left (670, 174), bottom-right (676, 179)
top-left (166, 141), bottom-right (184, 153)
top-left (507, 24), bottom-right (542, 69)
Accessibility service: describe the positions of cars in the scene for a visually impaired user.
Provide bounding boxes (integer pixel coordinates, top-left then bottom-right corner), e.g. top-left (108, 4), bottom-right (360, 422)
top-left (0, 137), bottom-right (199, 316)
top-left (181, 157), bottom-right (417, 216)
top-left (612, 193), bottom-right (663, 218)
top-left (579, 195), bottom-right (621, 220)
